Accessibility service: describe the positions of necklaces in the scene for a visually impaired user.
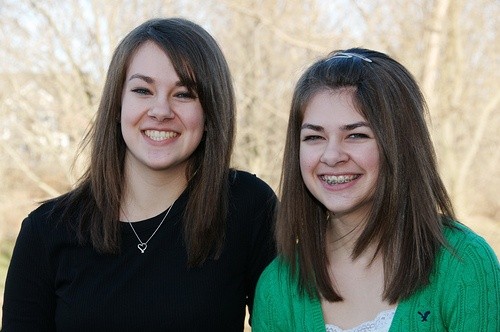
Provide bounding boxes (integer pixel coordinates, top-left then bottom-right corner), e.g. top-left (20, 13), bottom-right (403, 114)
top-left (117, 154), bottom-right (205, 254)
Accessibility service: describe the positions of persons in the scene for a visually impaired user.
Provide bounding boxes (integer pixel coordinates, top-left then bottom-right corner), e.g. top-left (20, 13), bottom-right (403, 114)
top-left (2, 16), bottom-right (280, 332)
top-left (250, 46), bottom-right (500, 332)
